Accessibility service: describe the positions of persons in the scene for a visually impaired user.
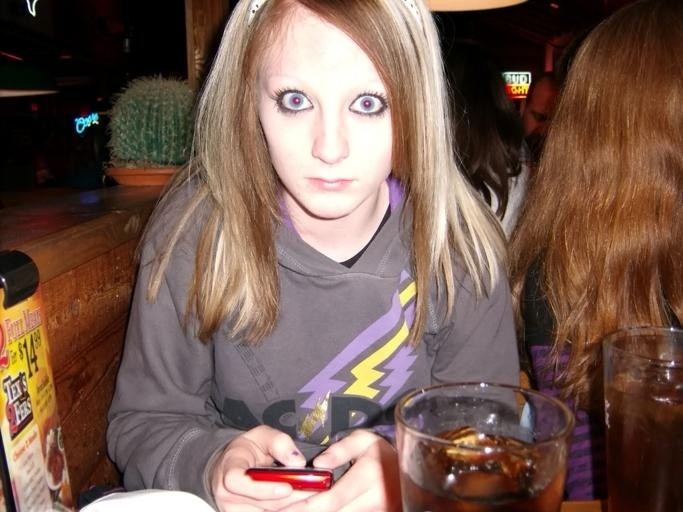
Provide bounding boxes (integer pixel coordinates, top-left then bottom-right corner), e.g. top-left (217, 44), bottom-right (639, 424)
top-left (444, 0), bottom-right (627, 243)
top-left (505, 1), bottom-right (683, 511)
top-left (105, 2), bottom-right (522, 512)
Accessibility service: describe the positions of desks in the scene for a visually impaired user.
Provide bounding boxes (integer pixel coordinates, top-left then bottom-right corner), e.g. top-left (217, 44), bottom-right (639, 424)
top-left (0, 185), bottom-right (157, 512)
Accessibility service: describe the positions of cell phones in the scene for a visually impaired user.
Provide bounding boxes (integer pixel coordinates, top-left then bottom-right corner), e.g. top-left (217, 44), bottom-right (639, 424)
top-left (245, 465), bottom-right (332, 493)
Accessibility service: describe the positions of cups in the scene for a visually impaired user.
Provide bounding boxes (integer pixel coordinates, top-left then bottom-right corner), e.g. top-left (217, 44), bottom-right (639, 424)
top-left (599, 327), bottom-right (683, 512)
top-left (392, 380), bottom-right (577, 512)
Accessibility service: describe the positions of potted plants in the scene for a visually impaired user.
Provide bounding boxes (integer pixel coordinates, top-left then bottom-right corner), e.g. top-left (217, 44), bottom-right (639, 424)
top-left (104, 74), bottom-right (199, 186)
